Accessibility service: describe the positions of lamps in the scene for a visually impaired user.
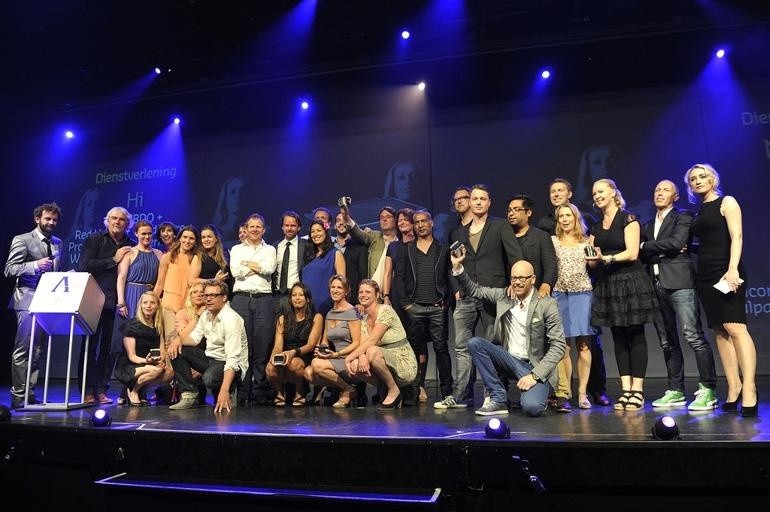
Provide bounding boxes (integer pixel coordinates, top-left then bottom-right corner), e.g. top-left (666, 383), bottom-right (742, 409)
top-left (651, 415), bottom-right (682, 443)
top-left (89, 406), bottom-right (115, 429)
top-left (483, 416), bottom-right (512, 439)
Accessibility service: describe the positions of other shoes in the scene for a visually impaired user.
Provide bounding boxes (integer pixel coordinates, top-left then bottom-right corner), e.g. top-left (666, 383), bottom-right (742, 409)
top-left (11, 395), bottom-right (30, 409)
top-left (95, 393), bottom-right (114, 404)
top-left (28, 395), bottom-right (43, 405)
top-left (116, 389), bottom-right (453, 409)
top-left (578, 392), bottom-right (592, 408)
top-left (84, 394), bottom-right (96, 403)
top-left (554, 397), bottom-right (572, 413)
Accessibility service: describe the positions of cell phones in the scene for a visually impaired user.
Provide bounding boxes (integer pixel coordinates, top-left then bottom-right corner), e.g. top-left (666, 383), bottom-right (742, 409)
top-left (713, 278), bottom-right (745, 294)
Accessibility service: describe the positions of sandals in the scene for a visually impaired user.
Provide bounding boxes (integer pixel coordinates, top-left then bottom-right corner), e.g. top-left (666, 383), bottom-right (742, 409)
top-left (613, 391), bottom-right (632, 411)
top-left (625, 391), bottom-right (646, 411)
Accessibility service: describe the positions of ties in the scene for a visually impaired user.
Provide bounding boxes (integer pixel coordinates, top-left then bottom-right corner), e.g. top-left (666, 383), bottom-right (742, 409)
top-left (43, 239), bottom-right (54, 272)
top-left (280, 242), bottom-right (291, 294)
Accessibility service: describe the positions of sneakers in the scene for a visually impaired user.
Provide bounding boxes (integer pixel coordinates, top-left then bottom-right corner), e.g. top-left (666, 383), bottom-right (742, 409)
top-left (433, 394), bottom-right (469, 408)
top-left (652, 390), bottom-right (687, 407)
top-left (482, 390), bottom-right (491, 408)
top-left (687, 382), bottom-right (720, 411)
top-left (475, 402), bottom-right (509, 415)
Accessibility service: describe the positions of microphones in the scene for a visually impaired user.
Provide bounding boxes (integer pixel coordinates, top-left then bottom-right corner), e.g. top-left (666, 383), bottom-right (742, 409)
top-left (38, 250), bottom-right (59, 272)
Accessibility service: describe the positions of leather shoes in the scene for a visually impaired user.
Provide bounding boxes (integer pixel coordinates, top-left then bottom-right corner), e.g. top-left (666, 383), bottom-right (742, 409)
top-left (587, 388), bottom-right (611, 406)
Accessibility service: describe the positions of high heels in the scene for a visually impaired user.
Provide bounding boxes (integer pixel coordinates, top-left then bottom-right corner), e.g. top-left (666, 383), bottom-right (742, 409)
top-left (740, 390), bottom-right (757, 416)
top-left (721, 389), bottom-right (742, 412)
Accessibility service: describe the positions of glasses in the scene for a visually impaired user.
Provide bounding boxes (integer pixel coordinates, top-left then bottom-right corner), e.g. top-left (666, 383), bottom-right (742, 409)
top-left (203, 294), bottom-right (223, 299)
top-left (453, 196), bottom-right (471, 202)
top-left (511, 275), bottom-right (533, 282)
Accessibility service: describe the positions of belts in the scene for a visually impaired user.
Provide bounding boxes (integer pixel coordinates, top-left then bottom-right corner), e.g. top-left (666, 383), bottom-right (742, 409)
top-left (236, 292), bottom-right (270, 297)
top-left (127, 281), bottom-right (154, 288)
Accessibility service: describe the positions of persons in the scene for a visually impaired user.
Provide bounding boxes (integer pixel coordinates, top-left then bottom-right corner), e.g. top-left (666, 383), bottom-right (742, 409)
top-left (638, 179), bottom-right (721, 411)
top-left (575, 139), bottom-right (629, 200)
top-left (5, 201), bottom-right (65, 411)
top-left (548, 202), bottom-right (604, 410)
top-left (68, 185), bottom-right (106, 238)
top-left (76, 182), bottom-right (560, 413)
top-left (209, 173), bottom-right (256, 242)
top-left (534, 178), bottom-right (613, 408)
top-left (682, 163), bottom-right (760, 418)
top-left (582, 176), bottom-right (657, 411)
top-left (449, 246), bottom-right (567, 416)
top-left (381, 158), bottom-right (425, 202)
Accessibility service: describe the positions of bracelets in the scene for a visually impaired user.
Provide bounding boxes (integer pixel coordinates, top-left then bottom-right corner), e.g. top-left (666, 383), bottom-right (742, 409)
top-left (602, 253), bottom-right (616, 265)
